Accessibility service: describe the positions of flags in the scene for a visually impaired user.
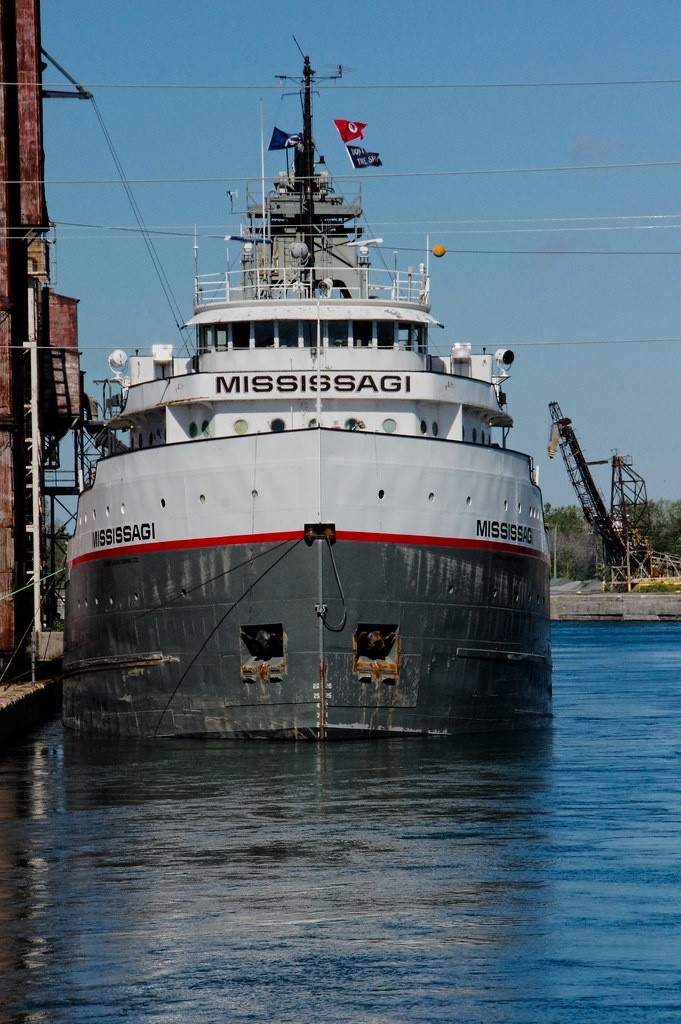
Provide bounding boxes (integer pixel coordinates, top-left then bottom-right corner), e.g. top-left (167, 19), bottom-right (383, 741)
top-left (346, 145), bottom-right (382, 168)
top-left (333, 119), bottom-right (367, 144)
top-left (267, 126), bottom-right (303, 151)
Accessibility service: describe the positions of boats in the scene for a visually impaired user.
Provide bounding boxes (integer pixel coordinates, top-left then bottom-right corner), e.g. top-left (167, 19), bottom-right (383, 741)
top-left (56, 52), bottom-right (552, 748)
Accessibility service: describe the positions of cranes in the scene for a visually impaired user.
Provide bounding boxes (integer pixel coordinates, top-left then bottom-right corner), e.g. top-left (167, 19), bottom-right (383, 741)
top-left (547, 398), bottom-right (662, 577)
